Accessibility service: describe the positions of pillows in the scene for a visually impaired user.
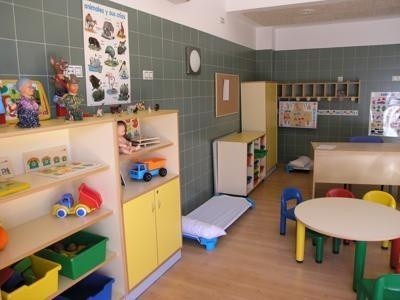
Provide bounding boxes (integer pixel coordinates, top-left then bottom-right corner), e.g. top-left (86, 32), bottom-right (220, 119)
top-left (182, 215), bottom-right (227, 239)
top-left (290, 155), bottom-right (311, 168)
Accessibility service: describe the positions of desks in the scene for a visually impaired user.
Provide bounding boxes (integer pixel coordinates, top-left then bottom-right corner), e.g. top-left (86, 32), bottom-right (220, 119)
top-left (310, 142), bottom-right (400, 199)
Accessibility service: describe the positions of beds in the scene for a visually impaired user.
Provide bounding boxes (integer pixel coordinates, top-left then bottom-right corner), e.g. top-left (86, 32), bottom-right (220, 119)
top-left (286, 160), bottom-right (313, 173)
top-left (182, 194), bottom-right (255, 251)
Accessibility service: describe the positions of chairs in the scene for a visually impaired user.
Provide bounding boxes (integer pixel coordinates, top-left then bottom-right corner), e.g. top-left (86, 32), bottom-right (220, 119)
top-left (280, 187), bottom-right (400, 300)
top-left (344, 137), bottom-right (384, 190)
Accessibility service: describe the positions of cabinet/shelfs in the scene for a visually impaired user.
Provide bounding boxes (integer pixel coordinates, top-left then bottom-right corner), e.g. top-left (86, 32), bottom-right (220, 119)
top-left (278, 82), bottom-right (360, 99)
top-left (0, 110), bottom-right (183, 300)
top-left (217, 132), bottom-right (266, 197)
top-left (241, 81), bottom-right (278, 181)
